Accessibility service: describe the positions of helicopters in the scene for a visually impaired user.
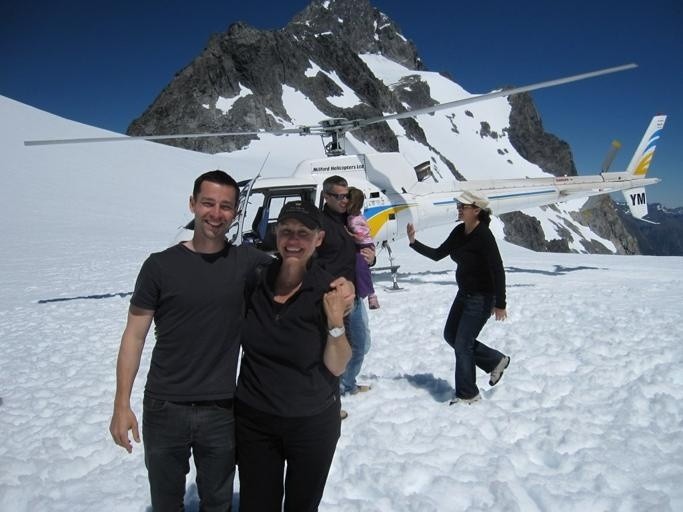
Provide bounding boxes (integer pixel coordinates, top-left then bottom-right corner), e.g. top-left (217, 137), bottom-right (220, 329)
top-left (22, 63), bottom-right (668, 290)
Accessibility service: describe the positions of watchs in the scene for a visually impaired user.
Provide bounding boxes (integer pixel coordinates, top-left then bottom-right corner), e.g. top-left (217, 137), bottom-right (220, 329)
top-left (326, 325), bottom-right (345, 338)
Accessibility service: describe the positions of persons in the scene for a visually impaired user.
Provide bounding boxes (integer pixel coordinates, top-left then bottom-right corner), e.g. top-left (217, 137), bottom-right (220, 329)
top-left (345, 186), bottom-right (379, 309)
top-left (311, 175), bottom-right (377, 423)
top-left (106, 171), bottom-right (360, 512)
top-left (406, 189), bottom-right (510, 408)
top-left (234, 199), bottom-right (352, 512)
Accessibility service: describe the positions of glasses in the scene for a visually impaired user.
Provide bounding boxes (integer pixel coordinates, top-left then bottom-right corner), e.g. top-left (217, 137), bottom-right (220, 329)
top-left (326, 191), bottom-right (349, 200)
top-left (457, 203), bottom-right (478, 209)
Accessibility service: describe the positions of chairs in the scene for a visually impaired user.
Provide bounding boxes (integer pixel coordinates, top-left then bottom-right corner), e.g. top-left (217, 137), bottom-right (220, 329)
top-left (242, 206), bottom-right (269, 246)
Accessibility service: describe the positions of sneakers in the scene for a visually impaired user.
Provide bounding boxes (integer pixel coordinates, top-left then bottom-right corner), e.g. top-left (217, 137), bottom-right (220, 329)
top-left (450, 393), bottom-right (481, 406)
top-left (367, 296), bottom-right (379, 308)
top-left (488, 356), bottom-right (510, 386)
top-left (350, 385), bottom-right (370, 395)
top-left (340, 410), bottom-right (348, 419)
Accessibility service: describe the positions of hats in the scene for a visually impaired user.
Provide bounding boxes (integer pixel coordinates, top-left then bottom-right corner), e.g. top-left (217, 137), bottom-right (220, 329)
top-left (453, 189), bottom-right (490, 209)
top-left (276, 200), bottom-right (320, 229)
